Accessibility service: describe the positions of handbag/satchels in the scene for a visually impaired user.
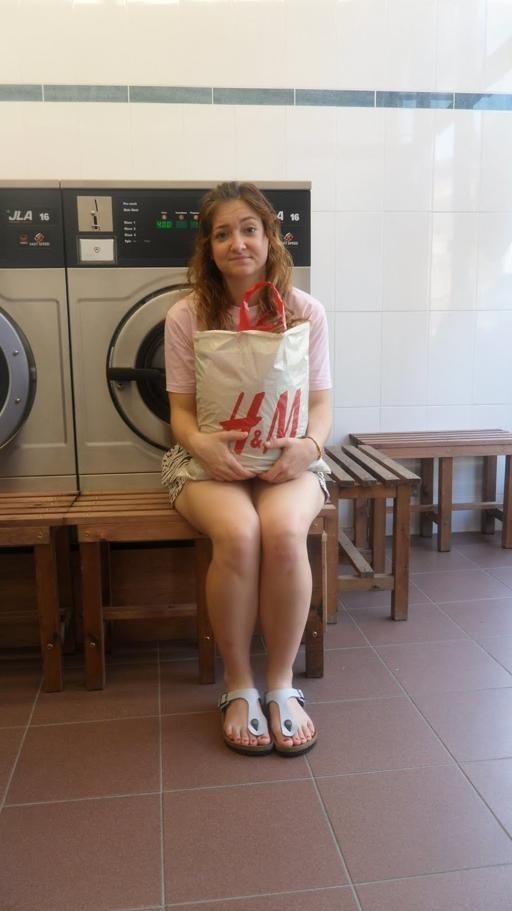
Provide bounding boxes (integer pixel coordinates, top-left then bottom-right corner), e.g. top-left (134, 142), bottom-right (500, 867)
top-left (173, 278), bottom-right (336, 485)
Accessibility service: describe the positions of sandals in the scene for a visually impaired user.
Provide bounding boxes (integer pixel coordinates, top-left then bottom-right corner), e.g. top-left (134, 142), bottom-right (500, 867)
top-left (264, 686), bottom-right (318, 758)
top-left (216, 686), bottom-right (275, 762)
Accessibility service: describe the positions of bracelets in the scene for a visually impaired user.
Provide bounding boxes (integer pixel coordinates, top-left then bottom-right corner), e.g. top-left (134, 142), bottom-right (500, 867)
top-left (300, 432), bottom-right (322, 462)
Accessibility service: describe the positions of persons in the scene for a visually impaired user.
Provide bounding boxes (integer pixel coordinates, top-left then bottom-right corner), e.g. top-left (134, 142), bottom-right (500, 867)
top-left (161, 176), bottom-right (336, 756)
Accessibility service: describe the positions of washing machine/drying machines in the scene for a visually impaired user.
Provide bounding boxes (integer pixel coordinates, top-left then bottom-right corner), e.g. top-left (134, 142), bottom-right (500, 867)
top-left (1, 180), bottom-right (76, 491)
top-left (67, 179), bottom-right (311, 492)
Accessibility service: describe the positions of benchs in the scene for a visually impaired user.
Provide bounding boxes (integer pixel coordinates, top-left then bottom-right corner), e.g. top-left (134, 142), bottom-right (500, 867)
top-left (320, 443), bottom-right (422, 624)
top-left (1, 490), bottom-right (81, 692)
top-left (347, 428), bottom-right (511, 552)
top-left (65, 490), bottom-right (337, 691)
